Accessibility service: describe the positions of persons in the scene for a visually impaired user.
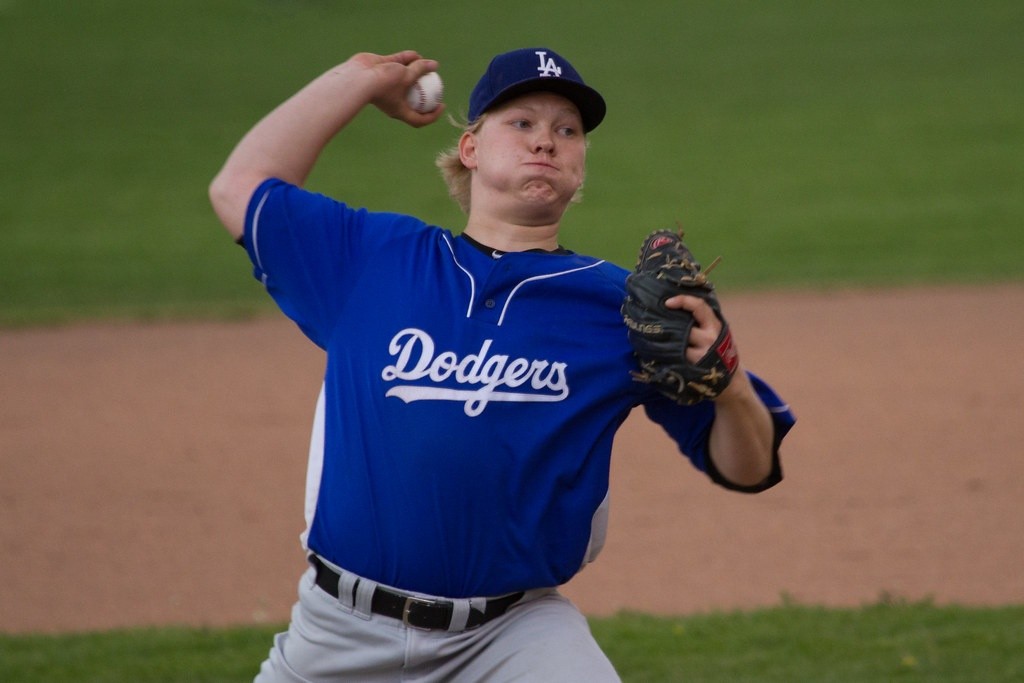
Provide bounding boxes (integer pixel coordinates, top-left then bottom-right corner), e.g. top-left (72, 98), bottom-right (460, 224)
top-left (209, 48), bottom-right (799, 682)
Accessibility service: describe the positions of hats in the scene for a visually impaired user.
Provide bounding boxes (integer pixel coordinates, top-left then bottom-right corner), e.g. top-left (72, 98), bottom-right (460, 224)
top-left (467, 47), bottom-right (606, 134)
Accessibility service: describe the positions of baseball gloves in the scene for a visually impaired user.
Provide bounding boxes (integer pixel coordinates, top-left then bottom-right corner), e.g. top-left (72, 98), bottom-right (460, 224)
top-left (619, 222), bottom-right (740, 410)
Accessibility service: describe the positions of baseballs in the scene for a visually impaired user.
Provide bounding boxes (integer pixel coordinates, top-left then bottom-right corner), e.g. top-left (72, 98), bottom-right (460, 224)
top-left (405, 71), bottom-right (444, 114)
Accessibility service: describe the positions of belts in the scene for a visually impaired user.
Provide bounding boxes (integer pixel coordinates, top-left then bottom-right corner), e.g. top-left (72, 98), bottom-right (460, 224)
top-left (310, 555), bottom-right (524, 632)
